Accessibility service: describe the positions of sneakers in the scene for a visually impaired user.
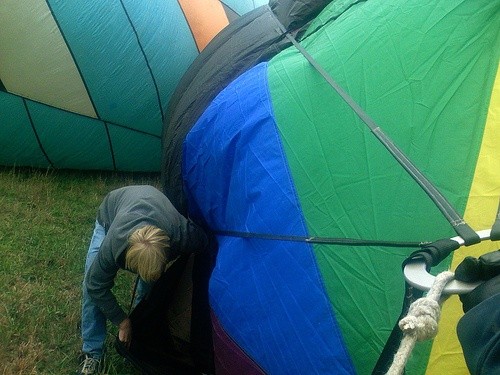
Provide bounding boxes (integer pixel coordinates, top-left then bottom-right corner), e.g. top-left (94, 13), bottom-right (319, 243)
top-left (76, 352), bottom-right (105, 375)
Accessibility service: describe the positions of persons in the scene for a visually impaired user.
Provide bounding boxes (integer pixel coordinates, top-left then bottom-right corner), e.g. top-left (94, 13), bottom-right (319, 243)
top-left (78, 183), bottom-right (193, 374)
